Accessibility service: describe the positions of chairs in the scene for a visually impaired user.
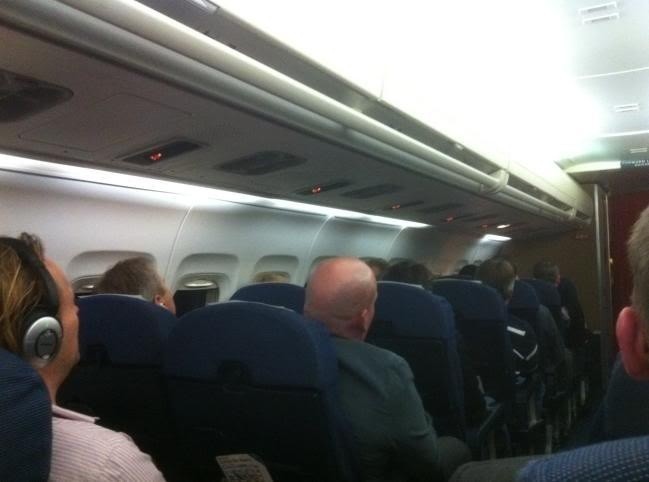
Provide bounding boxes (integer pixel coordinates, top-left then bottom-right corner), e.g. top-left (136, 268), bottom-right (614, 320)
top-left (2, 273), bottom-right (649, 481)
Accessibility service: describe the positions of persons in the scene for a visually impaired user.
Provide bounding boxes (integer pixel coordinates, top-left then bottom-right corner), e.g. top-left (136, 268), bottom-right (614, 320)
top-left (460, 258), bottom-right (603, 455)
top-left (301, 257), bottom-right (472, 482)
top-left (253, 257), bottom-right (434, 284)
top-left (0, 229), bottom-right (168, 481)
top-left (92, 252), bottom-right (176, 315)
top-left (512, 196), bottom-right (649, 482)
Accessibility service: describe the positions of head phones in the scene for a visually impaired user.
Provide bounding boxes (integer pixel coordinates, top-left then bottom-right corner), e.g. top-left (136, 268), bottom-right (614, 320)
top-left (0, 237), bottom-right (64, 368)
